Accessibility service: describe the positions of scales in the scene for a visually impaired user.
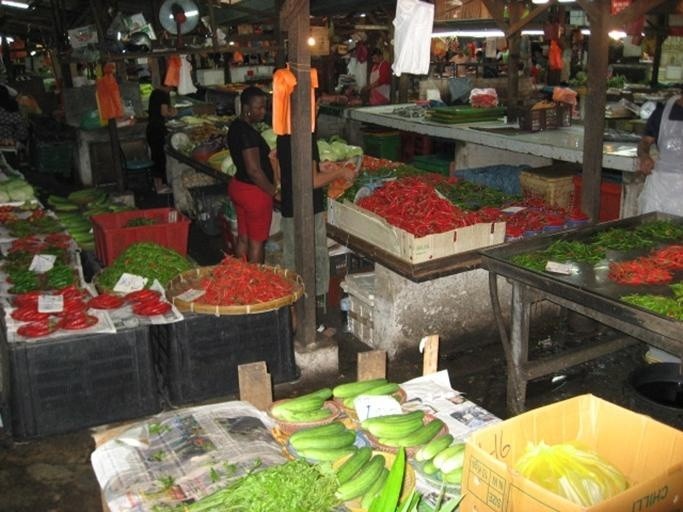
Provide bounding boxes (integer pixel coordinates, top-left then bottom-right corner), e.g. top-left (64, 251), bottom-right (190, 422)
top-left (640, 97), bottom-right (664, 121)
top-left (164, 120), bottom-right (190, 152)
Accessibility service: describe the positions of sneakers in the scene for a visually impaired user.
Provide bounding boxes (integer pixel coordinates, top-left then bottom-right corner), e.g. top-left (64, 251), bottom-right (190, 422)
top-left (151, 184), bottom-right (172, 195)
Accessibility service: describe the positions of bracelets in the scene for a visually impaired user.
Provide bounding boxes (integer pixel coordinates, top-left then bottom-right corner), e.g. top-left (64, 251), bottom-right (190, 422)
top-left (273, 188), bottom-right (279, 198)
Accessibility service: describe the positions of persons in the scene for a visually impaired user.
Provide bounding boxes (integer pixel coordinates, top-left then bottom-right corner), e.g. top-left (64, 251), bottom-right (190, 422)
top-left (277, 93), bottom-right (355, 337)
top-left (228, 84), bottom-right (281, 264)
top-left (0, 83), bottom-right (32, 174)
top-left (637, 81), bottom-right (683, 216)
top-left (146, 78), bottom-right (177, 195)
top-left (347, 46), bottom-right (370, 89)
top-left (360, 49), bottom-right (393, 102)
top-left (447, 39), bottom-right (549, 83)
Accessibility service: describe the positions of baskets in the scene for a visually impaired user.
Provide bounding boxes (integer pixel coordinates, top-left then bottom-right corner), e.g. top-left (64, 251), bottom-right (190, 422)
top-left (519, 99), bottom-right (572, 132)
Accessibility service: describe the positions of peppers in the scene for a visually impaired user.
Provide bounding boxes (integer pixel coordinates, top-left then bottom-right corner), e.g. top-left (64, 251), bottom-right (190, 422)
top-left (0, 205), bottom-right (289, 338)
top-left (356, 175), bottom-right (683, 322)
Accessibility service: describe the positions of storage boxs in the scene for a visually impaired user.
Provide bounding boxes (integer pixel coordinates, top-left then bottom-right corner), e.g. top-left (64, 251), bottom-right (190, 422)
top-left (460, 392), bottom-right (683, 511)
top-left (359, 127), bottom-right (576, 220)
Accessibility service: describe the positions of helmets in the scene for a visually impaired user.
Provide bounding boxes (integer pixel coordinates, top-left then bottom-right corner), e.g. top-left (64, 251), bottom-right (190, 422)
top-left (127, 32), bottom-right (153, 52)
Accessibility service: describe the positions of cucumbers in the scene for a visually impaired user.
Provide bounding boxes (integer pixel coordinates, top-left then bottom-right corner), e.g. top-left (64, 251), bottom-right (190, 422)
top-left (271, 378), bottom-right (464, 511)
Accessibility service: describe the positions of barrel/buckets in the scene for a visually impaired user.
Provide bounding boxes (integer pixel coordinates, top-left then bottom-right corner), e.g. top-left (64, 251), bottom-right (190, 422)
top-left (628, 360), bottom-right (683, 430)
